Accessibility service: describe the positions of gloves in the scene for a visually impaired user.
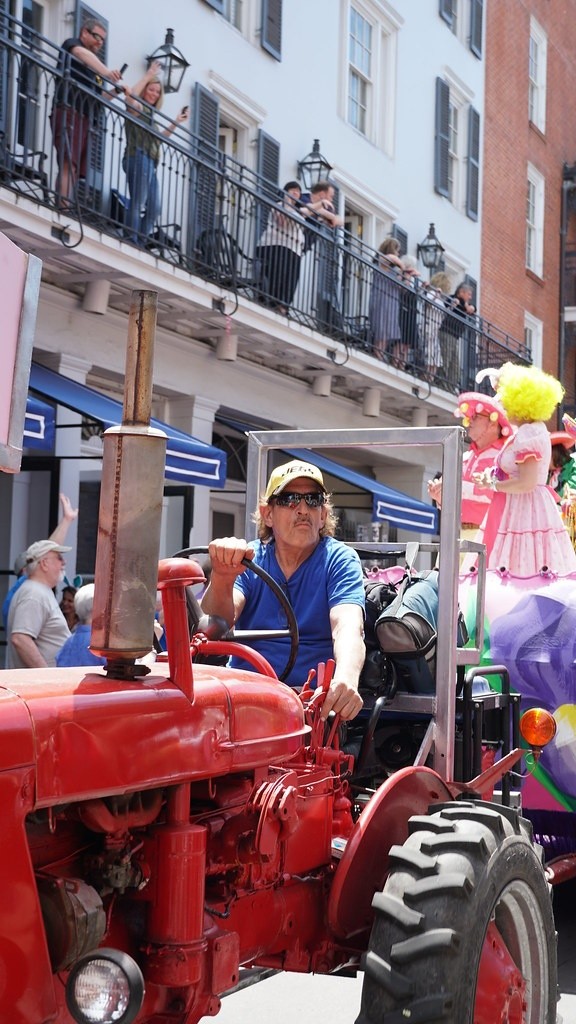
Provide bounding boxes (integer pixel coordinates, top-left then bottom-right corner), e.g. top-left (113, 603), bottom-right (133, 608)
top-left (472, 468), bottom-right (498, 493)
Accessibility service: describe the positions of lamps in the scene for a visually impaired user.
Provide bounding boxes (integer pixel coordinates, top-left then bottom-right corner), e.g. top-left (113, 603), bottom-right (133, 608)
top-left (83, 279), bottom-right (110, 314)
top-left (410, 409), bottom-right (428, 427)
top-left (363, 389), bottom-right (380, 416)
top-left (418, 222), bottom-right (446, 268)
top-left (216, 335), bottom-right (236, 361)
top-left (145, 28), bottom-right (190, 92)
top-left (298, 139), bottom-right (333, 191)
top-left (313, 376), bottom-right (332, 396)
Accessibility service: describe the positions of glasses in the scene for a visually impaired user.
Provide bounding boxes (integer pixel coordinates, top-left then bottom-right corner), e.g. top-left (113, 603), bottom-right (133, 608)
top-left (472, 412), bottom-right (490, 421)
top-left (276, 491), bottom-right (326, 508)
top-left (52, 556), bottom-right (63, 561)
top-left (86, 28), bottom-right (105, 44)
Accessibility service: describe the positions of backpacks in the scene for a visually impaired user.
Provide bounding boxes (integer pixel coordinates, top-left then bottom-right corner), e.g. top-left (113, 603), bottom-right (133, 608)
top-left (376, 570), bottom-right (469, 694)
top-left (363, 578), bottom-right (397, 642)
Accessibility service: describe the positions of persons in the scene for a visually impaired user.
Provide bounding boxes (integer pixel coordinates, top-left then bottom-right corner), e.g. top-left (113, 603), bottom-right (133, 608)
top-left (368, 237), bottom-right (477, 391)
top-left (55, 583), bottom-right (107, 667)
top-left (51, 18), bottom-right (132, 212)
top-left (427, 362), bottom-right (576, 590)
top-left (200, 460), bottom-right (366, 722)
top-left (62, 575), bottom-right (82, 630)
top-left (5, 540), bottom-right (72, 669)
top-left (3, 492), bottom-right (79, 628)
top-left (256, 181), bottom-right (341, 315)
top-left (122, 61), bottom-right (190, 249)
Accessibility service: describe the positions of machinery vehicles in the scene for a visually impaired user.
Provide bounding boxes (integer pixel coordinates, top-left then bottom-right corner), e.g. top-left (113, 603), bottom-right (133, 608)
top-left (0, 287), bottom-right (575, 1024)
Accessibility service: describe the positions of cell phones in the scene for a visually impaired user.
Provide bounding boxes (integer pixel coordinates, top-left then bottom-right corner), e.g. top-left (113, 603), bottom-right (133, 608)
top-left (181, 105), bottom-right (189, 118)
top-left (119, 64), bottom-right (129, 74)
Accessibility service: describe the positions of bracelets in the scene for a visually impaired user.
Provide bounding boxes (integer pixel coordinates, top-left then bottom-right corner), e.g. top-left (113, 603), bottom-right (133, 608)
top-left (115, 87), bottom-right (120, 93)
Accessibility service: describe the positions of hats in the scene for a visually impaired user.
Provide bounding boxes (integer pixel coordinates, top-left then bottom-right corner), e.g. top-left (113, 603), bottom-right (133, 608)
top-left (74, 583), bottom-right (95, 620)
top-left (25, 539), bottom-right (72, 563)
top-left (459, 391), bottom-right (513, 435)
top-left (550, 431), bottom-right (575, 450)
top-left (14, 553), bottom-right (27, 575)
top-left (265, 459), bottom-right (323, 502)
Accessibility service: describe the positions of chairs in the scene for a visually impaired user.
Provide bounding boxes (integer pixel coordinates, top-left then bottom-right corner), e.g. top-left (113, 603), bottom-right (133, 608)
top-left (110, 188), bottom-right (184, 264)
top-left (0, 131), bottom-right (50, 206)
top-left (322, 299), bottom-right (374, 351)
top-left (196, 230), bottom-right (264, 302)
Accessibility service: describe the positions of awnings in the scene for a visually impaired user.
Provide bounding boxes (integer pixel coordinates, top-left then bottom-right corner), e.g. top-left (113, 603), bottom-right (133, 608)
top-left (23, 360), bottom-right (226, 489)
top-left (210, 414), bottom-right (438, 534)
